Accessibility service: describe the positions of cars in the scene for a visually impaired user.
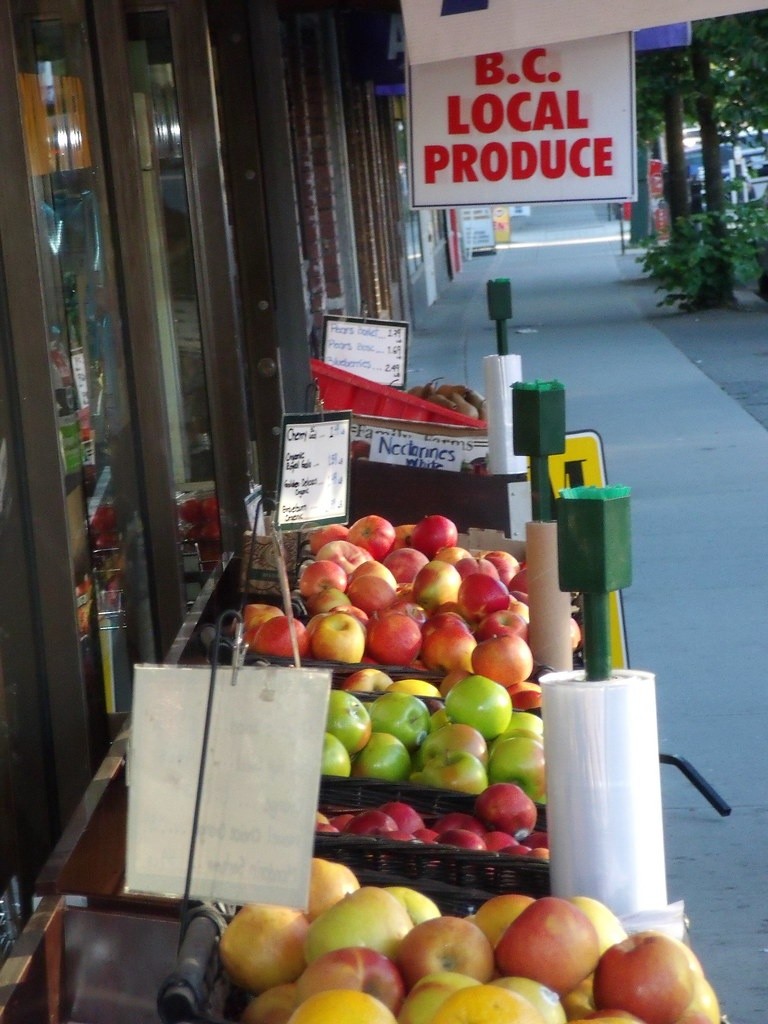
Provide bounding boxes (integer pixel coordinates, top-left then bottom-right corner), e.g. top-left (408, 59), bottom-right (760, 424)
top-left (661, 127), bottom-right (768, 211)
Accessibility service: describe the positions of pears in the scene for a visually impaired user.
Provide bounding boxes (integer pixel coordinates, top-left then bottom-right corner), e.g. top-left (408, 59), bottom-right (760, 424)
top-left (403, 375), bottom-right (487, 421)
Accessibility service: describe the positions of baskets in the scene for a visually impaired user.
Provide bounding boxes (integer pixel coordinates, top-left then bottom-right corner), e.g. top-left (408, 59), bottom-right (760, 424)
top-left (224, 521), bottom-right (584, 918)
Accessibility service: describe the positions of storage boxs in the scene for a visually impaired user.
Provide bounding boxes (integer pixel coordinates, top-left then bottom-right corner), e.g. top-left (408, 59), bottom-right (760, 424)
top-left (310, 359), bottom-right (485, 429)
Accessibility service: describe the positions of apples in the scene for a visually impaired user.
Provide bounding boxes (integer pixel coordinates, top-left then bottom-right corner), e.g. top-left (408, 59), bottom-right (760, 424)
top-left (90, 494), bottom-right (221, 567)
top-left (218, 857), bottom-right (723, 1024)
top-left (244, 513), bottom-right (582, 860)
